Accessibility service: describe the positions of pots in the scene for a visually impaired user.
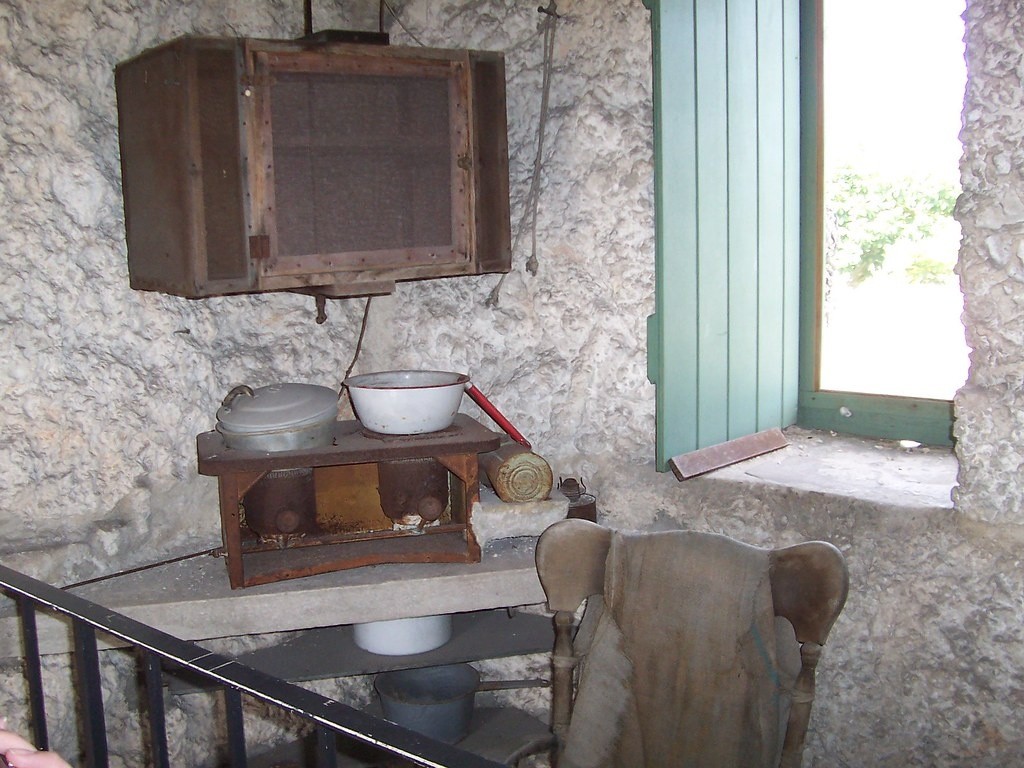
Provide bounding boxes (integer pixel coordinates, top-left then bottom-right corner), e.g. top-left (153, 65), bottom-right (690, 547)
top-left (216, 383), bottom-right (340, 452)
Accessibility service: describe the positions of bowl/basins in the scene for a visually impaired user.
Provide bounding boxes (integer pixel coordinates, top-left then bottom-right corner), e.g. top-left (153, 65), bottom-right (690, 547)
top-left (342, 369), bottom-right (470, 434)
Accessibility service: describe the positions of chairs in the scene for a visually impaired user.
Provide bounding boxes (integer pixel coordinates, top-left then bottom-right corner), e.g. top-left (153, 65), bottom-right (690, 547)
top-left (534, 518), bottom-right (849, 768)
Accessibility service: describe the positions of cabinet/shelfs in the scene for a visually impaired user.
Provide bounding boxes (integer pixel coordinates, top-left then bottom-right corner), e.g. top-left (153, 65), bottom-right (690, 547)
top-left (112, 35), bottom-right (512, 299)
top-left (0, 534), bottom-right (582, 768)
